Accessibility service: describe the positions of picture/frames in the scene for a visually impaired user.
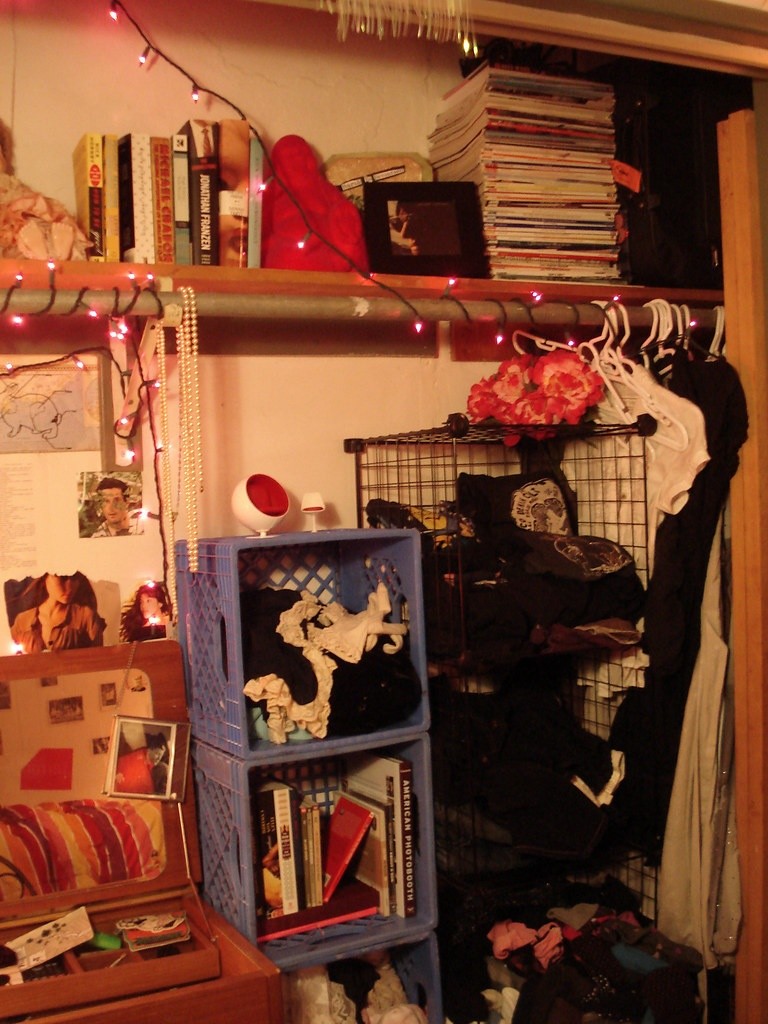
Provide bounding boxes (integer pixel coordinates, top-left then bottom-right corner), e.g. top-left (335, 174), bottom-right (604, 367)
top-left (363, 182), bottom-right (491, 279)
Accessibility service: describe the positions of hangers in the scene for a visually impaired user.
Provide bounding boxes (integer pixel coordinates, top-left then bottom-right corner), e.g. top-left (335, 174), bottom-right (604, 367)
top-left (511, 298), bottom-right (726, 469)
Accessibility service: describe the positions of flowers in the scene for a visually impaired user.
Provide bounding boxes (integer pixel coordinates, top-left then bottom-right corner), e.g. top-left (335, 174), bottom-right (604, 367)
top-left (466, 348), bottom-right (605, 448)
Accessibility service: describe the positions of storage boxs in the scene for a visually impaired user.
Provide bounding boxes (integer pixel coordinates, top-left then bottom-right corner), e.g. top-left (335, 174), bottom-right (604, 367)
top-left (275, 931), bottom-right (445, 1024)
top-left (172, 529), bottom-right (433, 762)
top-left (187, 735), bottom-right (439, 965)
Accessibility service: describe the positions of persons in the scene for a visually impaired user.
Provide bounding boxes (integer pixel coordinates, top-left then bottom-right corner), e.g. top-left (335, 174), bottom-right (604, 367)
top-left (11, 571), bottom-right (104, 653)
top-left (120, 580), bottom-right (175, 643)
top-left (114, 733), bottom-right (169, 796)
top-left (389, 201), bottom-right (459, 255)
top-left (88, 477), bottom-right (144, 538)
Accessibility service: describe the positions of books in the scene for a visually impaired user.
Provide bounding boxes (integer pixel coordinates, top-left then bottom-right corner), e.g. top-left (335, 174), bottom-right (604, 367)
top-left (71, 118), bottom-right (263, 269)
top-left (250, 753), bottom-right (417, 945)
top-left (426, 59), bottom-right (624, 288)
